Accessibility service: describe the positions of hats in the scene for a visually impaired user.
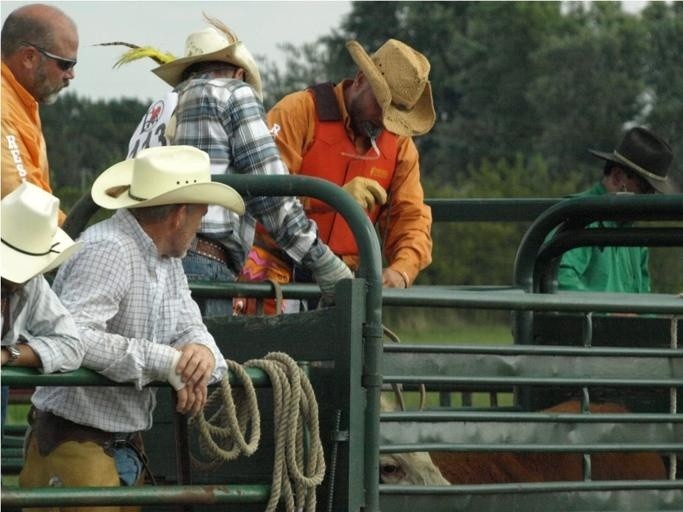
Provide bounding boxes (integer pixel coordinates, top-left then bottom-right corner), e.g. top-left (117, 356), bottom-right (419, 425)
top-left (92, 11), bottom-right (263, 103)
top-left (345, 39), bottom-right (436, 136)
top-left (1, 181), bottom-right (83, 284)
top-left (91, 145), bottom-right (246, 216)
top-left (587, 127), bottom-right (676, 195)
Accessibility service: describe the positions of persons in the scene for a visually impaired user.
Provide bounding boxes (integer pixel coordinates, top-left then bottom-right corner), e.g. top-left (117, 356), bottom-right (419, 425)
top-left (0, 5), bottom-right (77, 227)
top-left (0, 182), bottom-right (84, 453)
top-left (538, 127), bottom-right (679, 294)
top-left (231, 38), bottom-right (436, 318)
top-left (123, 26), bottom-right (352, 321)
top-left (22, 145), bottom-right (244, 511)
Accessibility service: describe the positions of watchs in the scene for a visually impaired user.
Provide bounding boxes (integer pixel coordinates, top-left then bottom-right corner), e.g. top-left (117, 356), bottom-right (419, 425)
top-left (1, 344), bottom-right (21, 366)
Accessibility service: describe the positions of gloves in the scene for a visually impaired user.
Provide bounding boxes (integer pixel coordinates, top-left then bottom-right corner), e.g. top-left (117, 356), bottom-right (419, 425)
top-left (303, 245), bottom-right (356, 305)
top-left (340, 176), bottom-right (386, 213)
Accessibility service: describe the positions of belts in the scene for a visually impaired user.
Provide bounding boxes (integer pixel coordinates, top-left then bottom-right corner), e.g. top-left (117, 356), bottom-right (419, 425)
top-left (188, 235), bottom-right (228, 264)
top-left (31, 408), bottom-right (139, 445)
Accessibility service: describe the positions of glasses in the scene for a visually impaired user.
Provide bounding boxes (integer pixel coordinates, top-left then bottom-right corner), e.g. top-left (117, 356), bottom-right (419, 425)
top-left (20, 41), bottom-right (77, 71)
